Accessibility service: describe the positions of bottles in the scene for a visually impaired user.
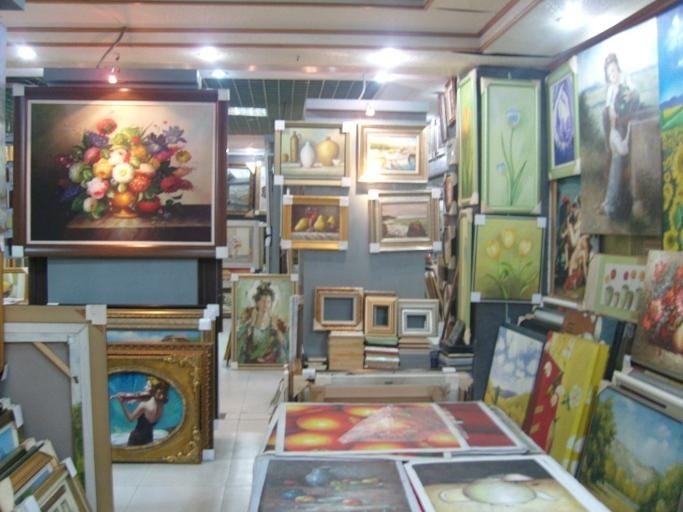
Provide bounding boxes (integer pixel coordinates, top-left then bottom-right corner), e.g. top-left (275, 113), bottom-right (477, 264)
top-left (603, 285), bottom-right (642, 312)
top-left (317, 136), bottom-right (339, 166)
top-left (300, 142), bottom-right (315, 167)
top-left (290, 131), bottom-right (298, 163)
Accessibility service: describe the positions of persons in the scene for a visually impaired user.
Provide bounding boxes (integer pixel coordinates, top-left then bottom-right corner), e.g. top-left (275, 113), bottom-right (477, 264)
top-left (107, 372), bottom-right (170, 448)
top-left (558, 197), bottom-right (590, 288)
top-left (234, 281), bottom-right (288, 363)
top-left (602, 52), bottom-right (626, 105)
top-left (599, 103), bottom-right (630, 217)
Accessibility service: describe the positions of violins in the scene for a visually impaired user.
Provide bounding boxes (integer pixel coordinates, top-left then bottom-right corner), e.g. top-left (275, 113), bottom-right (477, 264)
top-left (110, 392), bottom-right (151, 401)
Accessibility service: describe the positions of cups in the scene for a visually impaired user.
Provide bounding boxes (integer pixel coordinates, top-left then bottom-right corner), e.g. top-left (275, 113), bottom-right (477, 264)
top-left (279, 154), bottom-right (287, 163)
top-left (332, 160), bottom-right (340, 167)
